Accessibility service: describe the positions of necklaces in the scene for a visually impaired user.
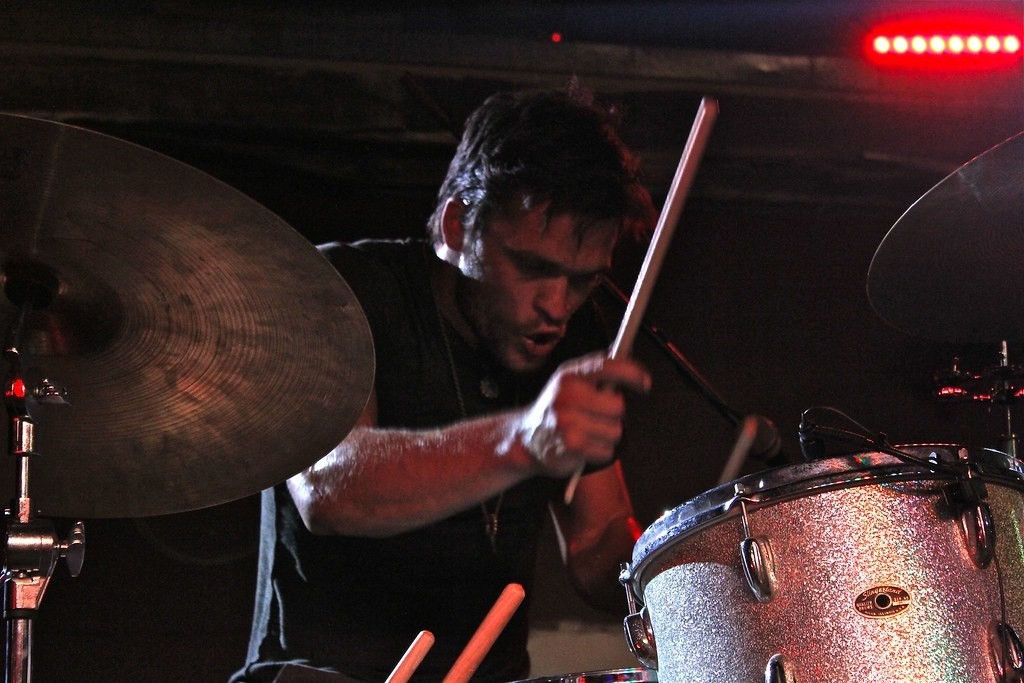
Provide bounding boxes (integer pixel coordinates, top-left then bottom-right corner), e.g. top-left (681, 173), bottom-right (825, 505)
top-left (430, 276), bottom-right (523, 539)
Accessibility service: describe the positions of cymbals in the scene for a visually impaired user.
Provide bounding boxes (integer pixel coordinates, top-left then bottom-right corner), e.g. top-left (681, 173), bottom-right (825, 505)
top-left (866, 130), bottom-right (1023, 344)
top-left (1, 112), bottom-right (376, 519)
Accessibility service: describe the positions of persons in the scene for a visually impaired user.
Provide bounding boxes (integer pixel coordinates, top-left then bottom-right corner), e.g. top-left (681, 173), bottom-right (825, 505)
top-left (223, 90), bottom-right (653, 682)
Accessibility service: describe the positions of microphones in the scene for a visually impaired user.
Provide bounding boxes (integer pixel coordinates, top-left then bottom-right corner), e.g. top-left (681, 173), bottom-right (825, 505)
top-left (798, 412), bottom-right (825, 461)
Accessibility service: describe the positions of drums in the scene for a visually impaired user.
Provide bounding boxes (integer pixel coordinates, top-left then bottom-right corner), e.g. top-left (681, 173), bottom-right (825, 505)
top-left (618, 444), bottom-right (1023, 683)
